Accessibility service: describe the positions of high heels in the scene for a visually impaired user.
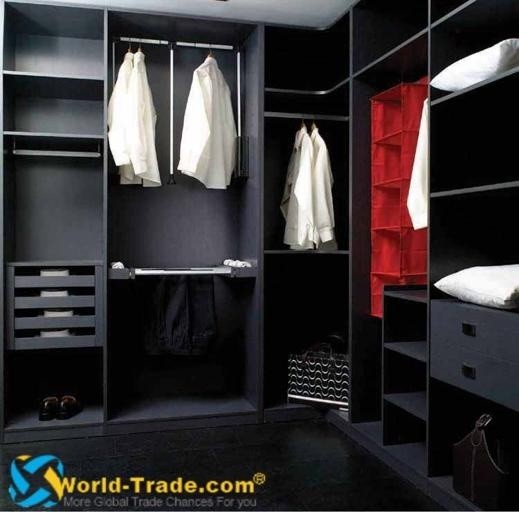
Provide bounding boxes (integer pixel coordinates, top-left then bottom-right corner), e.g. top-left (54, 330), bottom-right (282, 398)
top-left (287, 334), bottom-right (349, 410)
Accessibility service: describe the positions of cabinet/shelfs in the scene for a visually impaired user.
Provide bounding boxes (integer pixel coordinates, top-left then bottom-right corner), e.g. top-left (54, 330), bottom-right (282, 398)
top-left (0, 0), bottom-right (519, 511)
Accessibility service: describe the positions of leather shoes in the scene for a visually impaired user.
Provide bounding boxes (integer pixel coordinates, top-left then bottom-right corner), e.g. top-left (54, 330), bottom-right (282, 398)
top-left (39, 396), bottom-right (58, 421)
top-left (59, 396), bottom-right (82, 419)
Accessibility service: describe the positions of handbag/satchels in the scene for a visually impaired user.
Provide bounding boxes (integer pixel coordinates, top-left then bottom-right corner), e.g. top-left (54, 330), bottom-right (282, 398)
top-left (452, 408), bottom-right (512, 510)
